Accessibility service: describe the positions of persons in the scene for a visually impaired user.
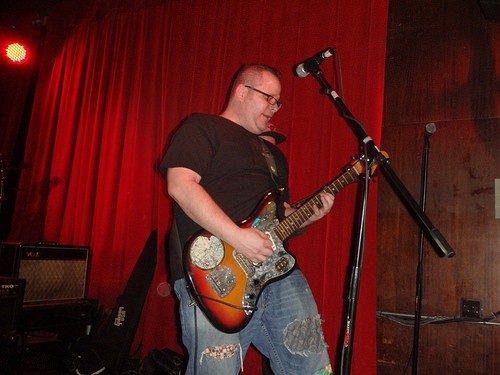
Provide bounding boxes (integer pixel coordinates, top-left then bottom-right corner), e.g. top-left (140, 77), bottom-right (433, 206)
top-left (153, 62), bottom-right (336, 375)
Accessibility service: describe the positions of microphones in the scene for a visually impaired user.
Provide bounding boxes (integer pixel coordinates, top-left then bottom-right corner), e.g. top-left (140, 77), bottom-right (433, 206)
top-left (292, 47), bottom-right (336, 78)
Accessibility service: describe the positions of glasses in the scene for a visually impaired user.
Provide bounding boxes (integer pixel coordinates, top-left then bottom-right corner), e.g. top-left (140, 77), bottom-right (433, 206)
top-left (245, 85), bottom-right (282, 109)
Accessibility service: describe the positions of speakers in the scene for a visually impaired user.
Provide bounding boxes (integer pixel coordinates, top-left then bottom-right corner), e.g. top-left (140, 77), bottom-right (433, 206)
top-left (0, 241), bottom-right (91, 303)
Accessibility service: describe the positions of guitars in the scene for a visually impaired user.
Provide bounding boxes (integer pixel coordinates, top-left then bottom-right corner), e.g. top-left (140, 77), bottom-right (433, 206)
top-left (183, 143), bottom-right (392, 334)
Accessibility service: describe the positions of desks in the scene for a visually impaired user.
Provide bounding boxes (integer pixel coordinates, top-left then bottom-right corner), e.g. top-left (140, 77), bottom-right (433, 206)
top-left (17, 298), bottom-right (96, 375)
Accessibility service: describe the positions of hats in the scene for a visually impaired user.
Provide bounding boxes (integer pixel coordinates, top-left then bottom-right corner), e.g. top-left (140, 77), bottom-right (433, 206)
top-left (256, 122), bottom-right (286, 145)
